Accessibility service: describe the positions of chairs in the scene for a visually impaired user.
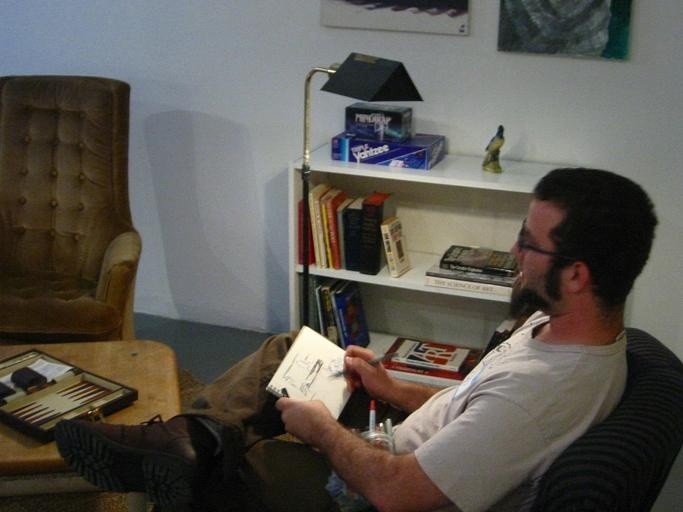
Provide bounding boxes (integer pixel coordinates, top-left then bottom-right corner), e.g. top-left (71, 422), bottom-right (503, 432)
top-left (1, 73), bottom-right (141, 334)
top-left (192, 325), bottom-right (681, 512)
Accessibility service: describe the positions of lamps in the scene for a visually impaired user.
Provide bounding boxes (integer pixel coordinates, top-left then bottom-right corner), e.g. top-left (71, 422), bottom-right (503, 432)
top-left (299, 45), bottom-right (424, 339)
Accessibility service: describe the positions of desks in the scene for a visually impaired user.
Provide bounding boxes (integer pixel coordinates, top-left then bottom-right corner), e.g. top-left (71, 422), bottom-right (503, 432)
top-left (0, 342), bottom-right (181, 512)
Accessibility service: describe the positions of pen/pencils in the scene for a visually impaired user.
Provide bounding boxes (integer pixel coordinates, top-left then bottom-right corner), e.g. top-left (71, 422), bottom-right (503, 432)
top-left (368, 400), bottom-right (395, 454)
top-left (334, 352), bottom-right (399, 377)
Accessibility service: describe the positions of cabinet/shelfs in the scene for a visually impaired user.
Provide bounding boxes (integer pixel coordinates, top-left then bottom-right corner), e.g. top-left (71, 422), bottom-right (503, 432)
top-left (288, 144), bottom-right (566, 402)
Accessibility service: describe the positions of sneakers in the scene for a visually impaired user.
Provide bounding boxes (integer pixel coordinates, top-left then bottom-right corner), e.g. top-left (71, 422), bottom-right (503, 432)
top-left (52, 414), bottom-right (222, 507)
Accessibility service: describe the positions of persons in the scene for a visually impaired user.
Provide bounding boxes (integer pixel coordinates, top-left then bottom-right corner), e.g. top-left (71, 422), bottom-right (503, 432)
top-left (55, 167), bottom-right (657, 512)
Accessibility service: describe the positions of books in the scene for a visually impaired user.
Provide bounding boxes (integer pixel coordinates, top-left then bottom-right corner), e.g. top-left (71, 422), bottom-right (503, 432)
top-left (298, 277), bottom-right (371, 351)
top-left (424, 243), bottom-right (522, 300)
top-left (379, 336), bottom-right (483, 385)
top-left (296, 183), bottom-right (413, 279)
top-left (266, 324), bottom-right (366, 428)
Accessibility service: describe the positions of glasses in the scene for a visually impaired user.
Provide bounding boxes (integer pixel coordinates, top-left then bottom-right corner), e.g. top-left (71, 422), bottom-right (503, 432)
top-left (516, 218), bottom-right (559, 259)
